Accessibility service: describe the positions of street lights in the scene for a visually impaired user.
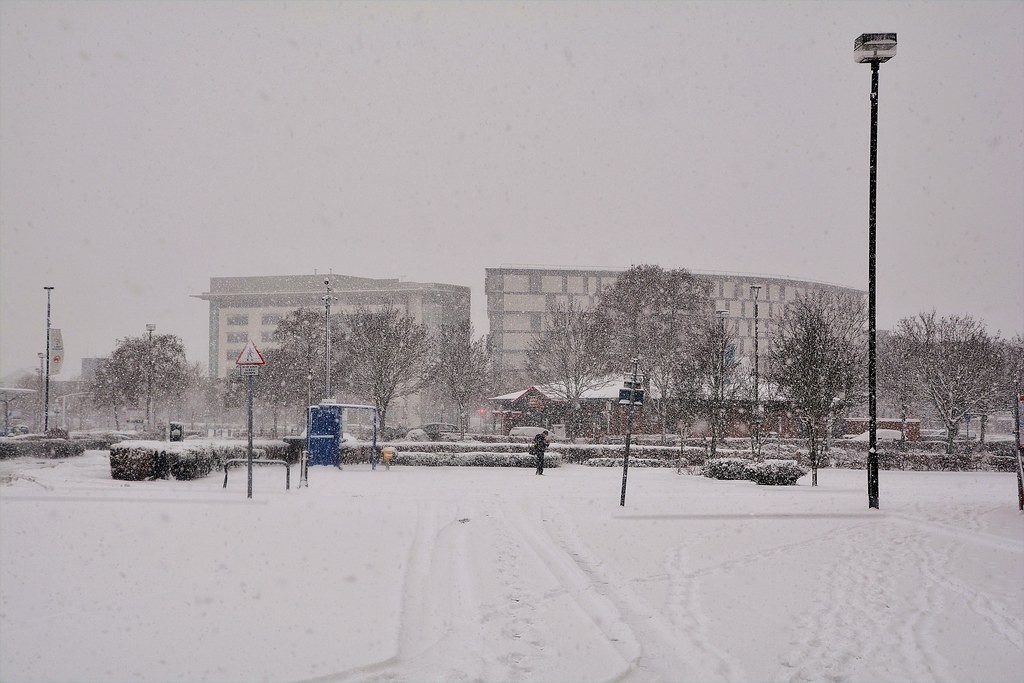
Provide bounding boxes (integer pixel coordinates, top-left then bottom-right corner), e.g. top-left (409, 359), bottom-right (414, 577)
top-left (144, 323), bottom-right (157, 426)
top-left (750, 285), bottom-right (765, 432)
top-left (45, 286), bottom-right (54, 432)
top-left (712, 311), bottom-right (733, 404)
top-left (852, 30), bottom-right (900, 506)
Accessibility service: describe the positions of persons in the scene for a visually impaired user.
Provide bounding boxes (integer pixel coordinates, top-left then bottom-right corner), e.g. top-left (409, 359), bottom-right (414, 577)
top-left (533, 430), bottom-right (550, 475)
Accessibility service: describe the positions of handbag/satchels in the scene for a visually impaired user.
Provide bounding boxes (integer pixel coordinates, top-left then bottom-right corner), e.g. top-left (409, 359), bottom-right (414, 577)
top-left (529, 446), bottom-right (536, 454)
